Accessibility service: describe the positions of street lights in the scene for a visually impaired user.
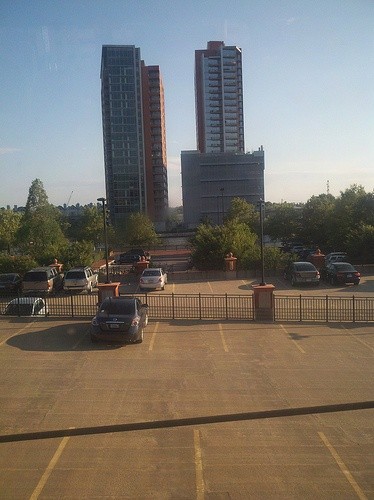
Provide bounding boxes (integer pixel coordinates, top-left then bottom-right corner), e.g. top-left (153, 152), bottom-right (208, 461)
top-left (97, 198), bottom-right (112, 284)
top-left (257, 199), bottom-right (266, 285)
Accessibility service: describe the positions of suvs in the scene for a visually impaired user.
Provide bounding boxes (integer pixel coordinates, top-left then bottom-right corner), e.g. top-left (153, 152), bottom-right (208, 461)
top-left (63, 266), bottom-right (98, 293)
top-left (22, 267), bottom-right (64, 297)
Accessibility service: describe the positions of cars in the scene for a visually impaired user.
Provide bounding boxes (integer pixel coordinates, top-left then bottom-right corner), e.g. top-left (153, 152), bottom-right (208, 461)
top-left (0, 273), bottom-right (23, 296)
top-left (2, 297), bottom-right (48, 317)
top-left (323, 263), bottom-right (360, 286)
top-left (91, 296), bottom-right (149, 343)
top-left (283, 262), bottom-right (320, 287)
top-left (139, 268), bottom-right (168, 290)
